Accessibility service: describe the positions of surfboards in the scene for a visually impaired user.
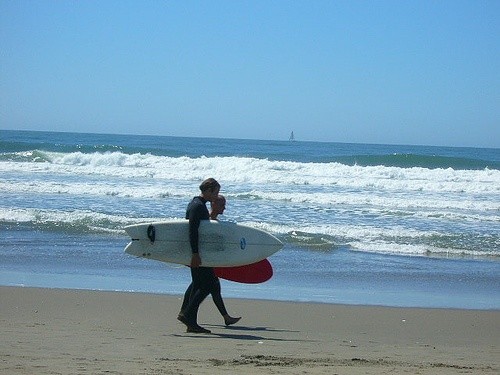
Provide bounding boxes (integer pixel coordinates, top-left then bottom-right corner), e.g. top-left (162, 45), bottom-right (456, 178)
top-left (185, 258), bottom-right (273, 283)
top-left (124, 220), bottom-right (284, 267)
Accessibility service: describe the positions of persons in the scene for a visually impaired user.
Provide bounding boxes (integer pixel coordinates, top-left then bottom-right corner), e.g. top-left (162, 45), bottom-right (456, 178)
top-left (185, 178), bottom-right (221, 333)
top-left (176, 195), bottom-right (242, 326)
